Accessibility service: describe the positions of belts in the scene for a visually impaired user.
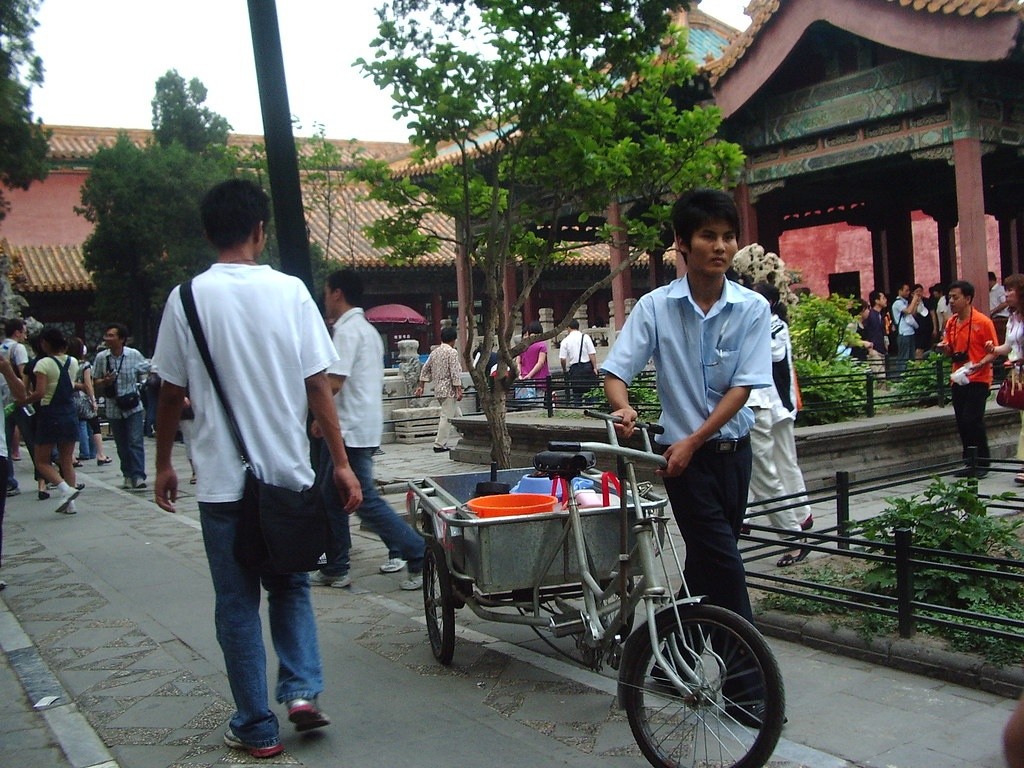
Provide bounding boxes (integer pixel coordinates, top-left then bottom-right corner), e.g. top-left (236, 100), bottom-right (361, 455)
top-left (701, 432), bottom-right (750, 453)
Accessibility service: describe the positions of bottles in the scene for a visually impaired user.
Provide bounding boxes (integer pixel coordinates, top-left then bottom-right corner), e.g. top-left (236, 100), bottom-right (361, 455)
top-left (22, 404), bottom-right (35, 416)
top-left (4, 399), bottom-right (16, 418)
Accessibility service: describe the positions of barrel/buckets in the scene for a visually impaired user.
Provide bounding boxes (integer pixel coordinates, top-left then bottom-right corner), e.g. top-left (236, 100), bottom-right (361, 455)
top-left (460, 493), bottom-right (559, 518)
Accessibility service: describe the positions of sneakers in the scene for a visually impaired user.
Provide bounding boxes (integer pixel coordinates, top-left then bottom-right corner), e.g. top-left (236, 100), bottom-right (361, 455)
top-left (222, 727), bottom-right (285, 759)
top-left (398, 568), bottom-right (437, 590)
top-left (307, 569), bottom-right (343, 585)
top-left (380, 557), bottom-right (407, 572)
top-left (54, 487), bottom-right (80, 514)
top-left (286, 695), bottom-right (331, 731)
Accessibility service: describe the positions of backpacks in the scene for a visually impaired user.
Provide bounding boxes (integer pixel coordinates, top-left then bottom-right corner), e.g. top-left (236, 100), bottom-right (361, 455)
top-left (882, 299), bottom-right (905, 338)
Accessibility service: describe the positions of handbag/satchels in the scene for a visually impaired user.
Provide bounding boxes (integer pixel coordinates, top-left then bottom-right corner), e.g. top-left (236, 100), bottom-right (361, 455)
top-left (115, 392), bottom-right (139, 411)
top-left (997, 378), bottom-right (1024, 410)
top-left (243, 467), bottom-right (329, 571)
top-left (74, 391), bottom-right (95, 421)
top-left (571, 367), bottom-right (598, 392)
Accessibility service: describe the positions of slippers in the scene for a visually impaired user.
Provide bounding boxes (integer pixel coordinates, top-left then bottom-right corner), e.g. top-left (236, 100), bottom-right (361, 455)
top-left (73, 461), bottom-right (84, 467)
top-left (97, 456), bottom-right (112, 466)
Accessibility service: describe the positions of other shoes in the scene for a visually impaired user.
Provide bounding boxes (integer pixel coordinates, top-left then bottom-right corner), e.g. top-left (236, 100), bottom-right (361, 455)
top-left (649, 667), bottom-right (720, 705)
top-left (7, 488), bottom-right (20, 495)
top-left (38, 490), bottom-right (50, 500)
top-left (76, 456), bottom-right (89, 460)
top-left (122, 476), bottom-right (132, 488)
top-left (957, 465), bottom-right (990, 478)
top-left (801, 514), bottom-right (813, 529)
top-left (740, 527), bottom-right (751, 535)
top-left (724, 702), bottom-right (787, 727)
top-left (777, 546), bottom-right (809, 567)
top-left (134, 476), bottom-right (147, 488)
top-left (1014, 471), bottom-right (1024, 483)
top-left (189, 473), bottom-right (197, 485)
top-left (434, 446), bottom-right (450, 452)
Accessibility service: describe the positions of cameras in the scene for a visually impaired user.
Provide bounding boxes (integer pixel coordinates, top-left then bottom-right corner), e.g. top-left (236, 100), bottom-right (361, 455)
top-left (952, 352), bottom-right (969, 363)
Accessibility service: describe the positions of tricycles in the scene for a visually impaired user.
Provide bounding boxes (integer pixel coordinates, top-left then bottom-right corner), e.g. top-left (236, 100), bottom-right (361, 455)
top-left (407, 411), bottom-right (785, 768)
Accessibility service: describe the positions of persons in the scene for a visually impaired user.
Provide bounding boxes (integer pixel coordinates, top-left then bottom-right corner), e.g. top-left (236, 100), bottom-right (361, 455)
top-left (413, 327), bottom-right (463, 453)
top-left (842, 272), bottom-right (1024, 484)
top-left (306, 269), bottom-right (425, 591)
top-left (598, 190), bottom-right (789, 730)
top-left (473, 341), bottom-right (510, 376)
top-left (559, 318), bottom-right (598, 406)
top-left (1, 318), bottom-right (197, 591)
top-left (515, 323), bottom-right (555, 410)
top-left (151, 178), bottom-right (363, 760)
top-left (739, 283), bottom-right (814, 567)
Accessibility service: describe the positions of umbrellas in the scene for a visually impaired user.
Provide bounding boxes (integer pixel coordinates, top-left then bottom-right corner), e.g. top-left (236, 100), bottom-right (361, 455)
top-left (364, 304), bottom-right (431, 326)
